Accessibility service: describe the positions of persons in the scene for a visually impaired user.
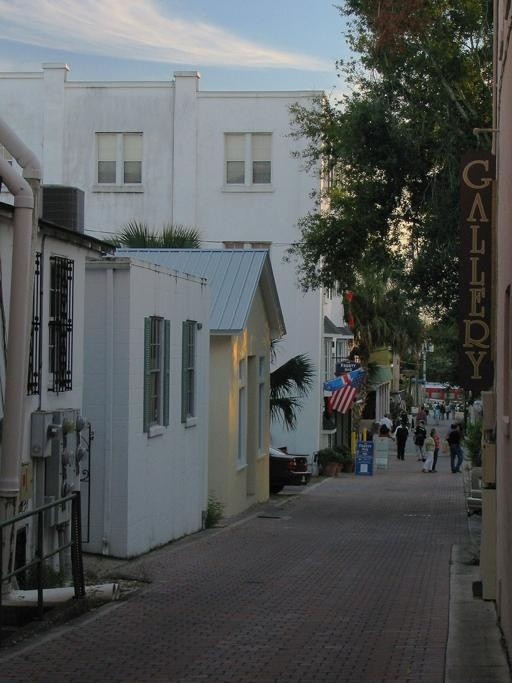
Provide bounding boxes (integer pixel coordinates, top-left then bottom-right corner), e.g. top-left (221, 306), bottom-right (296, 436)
top-left (379, 398), bottom-right (483, 473)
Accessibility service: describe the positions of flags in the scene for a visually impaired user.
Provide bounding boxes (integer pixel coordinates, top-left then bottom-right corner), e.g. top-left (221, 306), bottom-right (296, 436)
top-left (329, 378), bottom-right (362, 414)
top-left (323, 368), bottom-right (366, 391)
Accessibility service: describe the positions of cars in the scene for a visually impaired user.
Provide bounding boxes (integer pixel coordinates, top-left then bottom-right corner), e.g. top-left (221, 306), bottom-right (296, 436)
top-left (269, 447), bottom-right (312, 494)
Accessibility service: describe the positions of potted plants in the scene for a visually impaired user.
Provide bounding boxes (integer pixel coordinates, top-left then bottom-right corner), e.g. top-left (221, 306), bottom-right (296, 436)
top-left (313, 444), bottom-right (358, 477)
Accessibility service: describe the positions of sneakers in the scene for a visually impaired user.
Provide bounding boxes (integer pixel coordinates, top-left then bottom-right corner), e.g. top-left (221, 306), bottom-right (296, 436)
top-left (418, 458), bottom-right (425, 462)
top-left (423, 469), bottom-right (436, 473)
